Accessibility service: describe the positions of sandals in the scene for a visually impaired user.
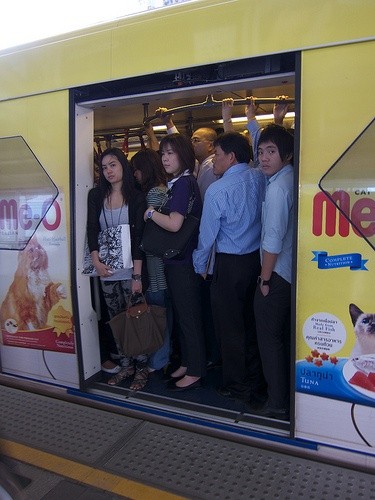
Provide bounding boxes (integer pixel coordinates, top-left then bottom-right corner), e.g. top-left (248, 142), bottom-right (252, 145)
top-left (108, 364), bottom-right (135, 386)
top-left (130, 365), bottom-right (149, 390)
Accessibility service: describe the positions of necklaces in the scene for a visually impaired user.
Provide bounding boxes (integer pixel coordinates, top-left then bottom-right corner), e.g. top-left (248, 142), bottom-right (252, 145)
top-left (108, 194), bottom-right (123, 208)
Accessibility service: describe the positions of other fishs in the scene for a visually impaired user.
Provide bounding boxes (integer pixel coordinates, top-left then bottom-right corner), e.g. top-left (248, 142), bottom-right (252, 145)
top-left (351, 357), bottom-right (375, 374)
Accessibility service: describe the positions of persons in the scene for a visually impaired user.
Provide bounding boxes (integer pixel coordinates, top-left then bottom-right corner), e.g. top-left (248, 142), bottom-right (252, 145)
top-left (87, 94), bottom-right (294, 420)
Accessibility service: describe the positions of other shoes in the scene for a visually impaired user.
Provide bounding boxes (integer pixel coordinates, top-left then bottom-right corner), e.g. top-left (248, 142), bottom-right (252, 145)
top-left (102, 365), bottom-right (122, 373)
top-left (168, 375), bottom-right (201, 392)
top-left (162, 366), bottom-right (185, 382)
top-left (149, 366), bottom-right (162, 373)
top-left (205, 363), bottom-right (222, 371)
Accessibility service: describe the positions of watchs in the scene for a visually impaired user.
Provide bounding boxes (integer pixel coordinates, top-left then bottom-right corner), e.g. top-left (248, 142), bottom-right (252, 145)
top-left (257, 276), bottom-right (270, 287)
top-left (132, 274), bottom-right (141, 281)
top-left (146, 209), bottom-right (155, 218)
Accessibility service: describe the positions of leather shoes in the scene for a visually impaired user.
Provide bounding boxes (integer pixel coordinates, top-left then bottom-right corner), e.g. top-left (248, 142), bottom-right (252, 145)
top-left (216, 386), bottom-right (253, 401)
top-left (246, 403), bottom-right (289, 418)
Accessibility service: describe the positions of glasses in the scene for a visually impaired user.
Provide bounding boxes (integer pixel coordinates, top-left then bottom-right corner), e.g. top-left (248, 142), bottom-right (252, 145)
top-left (190, 139), bottom-right (209, 143)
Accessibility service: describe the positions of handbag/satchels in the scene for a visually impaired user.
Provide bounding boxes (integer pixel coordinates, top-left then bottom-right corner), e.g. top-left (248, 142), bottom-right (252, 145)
top-left (139, 176), bottom-right (200, 259)
top-left (81, 224), bottom-right (134, 276)
top-left (107, 292), bottom-right (167, 356)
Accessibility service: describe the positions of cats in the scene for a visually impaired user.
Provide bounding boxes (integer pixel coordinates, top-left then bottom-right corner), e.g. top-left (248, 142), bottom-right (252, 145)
top-left (349, 303), bottom-right (375, 357)
top-left (0, 234), bottom-right (68, 334)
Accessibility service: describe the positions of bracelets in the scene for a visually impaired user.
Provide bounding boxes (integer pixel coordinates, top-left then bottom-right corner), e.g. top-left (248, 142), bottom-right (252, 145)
top-left (248, 117), bottom-right (256, 122)
top-left (223, 118), bottom-right (231, 122)
